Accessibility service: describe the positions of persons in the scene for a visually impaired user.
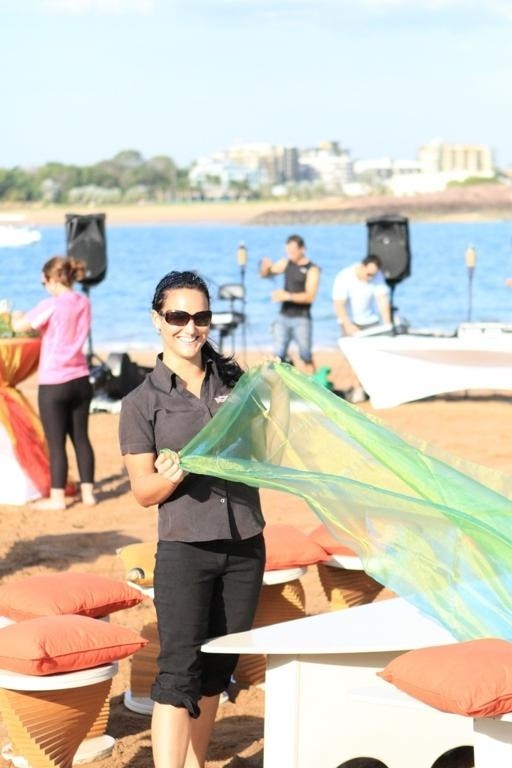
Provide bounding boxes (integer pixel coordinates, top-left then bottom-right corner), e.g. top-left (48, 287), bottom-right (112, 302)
top-left (117, 270), bottom-right (267, 768)
top-left (261, 232), bottom-right (322, 380)
top-left (12, 257), bottom-right (97, 510)
top-left (331, 253), bottom-right (393, 404)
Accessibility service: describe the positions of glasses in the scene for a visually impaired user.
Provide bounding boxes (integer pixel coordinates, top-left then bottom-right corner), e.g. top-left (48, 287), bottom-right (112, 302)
top-left (158, 309), bottom-right (212, 326)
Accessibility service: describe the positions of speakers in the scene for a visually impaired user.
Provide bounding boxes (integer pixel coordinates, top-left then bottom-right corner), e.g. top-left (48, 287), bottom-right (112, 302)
top-left (65, 212), bottom-right (109, 286)
top-left (366, 215), bottom-right (411, 284)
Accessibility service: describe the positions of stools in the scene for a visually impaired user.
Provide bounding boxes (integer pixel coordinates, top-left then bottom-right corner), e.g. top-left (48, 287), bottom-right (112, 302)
top-left (1, 663), bottom-right (117, 768)
top-left (227, 557), bottom-right (384, 687)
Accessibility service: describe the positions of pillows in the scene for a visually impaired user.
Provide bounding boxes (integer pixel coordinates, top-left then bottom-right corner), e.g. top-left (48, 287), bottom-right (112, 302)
top-left (0, 565), bottom-right (150, 673)
top-left (262, 520), bottom-right (360, 567)
top-left (371, 638), bottom-right (512, 720)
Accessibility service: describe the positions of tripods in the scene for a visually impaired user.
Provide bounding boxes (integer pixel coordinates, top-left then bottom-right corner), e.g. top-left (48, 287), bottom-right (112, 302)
top-left (81, 288), bottom-right (106, 369)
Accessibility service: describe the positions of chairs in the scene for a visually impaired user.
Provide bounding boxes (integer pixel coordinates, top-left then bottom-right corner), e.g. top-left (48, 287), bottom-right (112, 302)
top-left (209, 284), bottom-right (248, 353)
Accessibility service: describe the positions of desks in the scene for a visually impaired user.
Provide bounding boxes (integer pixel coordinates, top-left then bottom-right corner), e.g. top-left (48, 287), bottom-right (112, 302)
top-left (337, 335), bottom-right (511, 410)
top-left (200, 579), bottom-right (512, 762)
